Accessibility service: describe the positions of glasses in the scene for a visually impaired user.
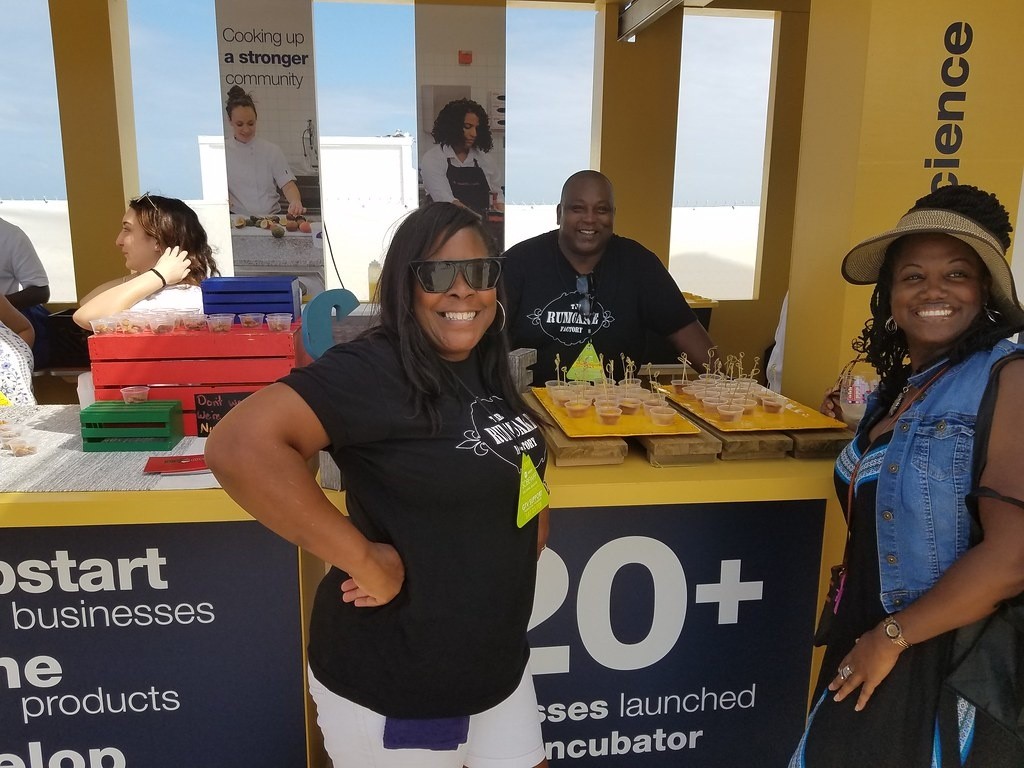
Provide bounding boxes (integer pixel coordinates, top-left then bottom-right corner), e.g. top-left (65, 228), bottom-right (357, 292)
top-left (410, 258), bottom-right (501, 292)
top-left (137, 192), bottom-right (158, 212)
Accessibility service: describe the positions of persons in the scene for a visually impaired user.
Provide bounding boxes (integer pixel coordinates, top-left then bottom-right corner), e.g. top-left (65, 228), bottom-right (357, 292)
top-left (787, 185), bottom-right (1024, 768)
top-left (73, 190), bottom-right (221, 331)
top-left (420, 97), bottom-right (505, 218)
top-left (0, 217), bottom-right (50, 406)
top-left (500, 171), bottom-right (734, 388)
top-left (224, 86), bottom-right (308, 217)
top-left (204, 201), bottom-right (549, 768)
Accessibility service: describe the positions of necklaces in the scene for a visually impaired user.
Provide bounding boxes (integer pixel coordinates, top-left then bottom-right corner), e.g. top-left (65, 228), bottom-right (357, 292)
top-left (889, 374), bottom-right (913, 417)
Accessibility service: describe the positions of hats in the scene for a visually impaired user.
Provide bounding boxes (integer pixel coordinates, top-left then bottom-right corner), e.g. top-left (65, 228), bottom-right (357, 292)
top-left (844, 208), bottom-right (1024, 325)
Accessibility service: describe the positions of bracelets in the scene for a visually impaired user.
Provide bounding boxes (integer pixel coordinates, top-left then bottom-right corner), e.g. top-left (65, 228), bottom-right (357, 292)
top-left (150, 269), bottom-right (166, 288)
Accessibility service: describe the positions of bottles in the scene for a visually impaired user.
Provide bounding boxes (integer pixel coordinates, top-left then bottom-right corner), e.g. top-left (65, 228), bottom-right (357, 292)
top-left (368, 259), bottom-right (382, 303)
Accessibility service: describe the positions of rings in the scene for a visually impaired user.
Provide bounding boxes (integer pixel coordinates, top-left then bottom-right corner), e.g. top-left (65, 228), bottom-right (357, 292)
top-left (840, 665), bottom-right (853, 679)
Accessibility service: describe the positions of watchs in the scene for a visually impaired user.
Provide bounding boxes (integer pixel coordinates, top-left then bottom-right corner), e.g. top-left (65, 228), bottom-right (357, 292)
top-left (884, 614), bottom-right (912, 648)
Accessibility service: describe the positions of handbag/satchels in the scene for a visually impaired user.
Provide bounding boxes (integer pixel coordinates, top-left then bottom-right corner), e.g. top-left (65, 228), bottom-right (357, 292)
top-left (939, 590), bottom-right (1024, 736)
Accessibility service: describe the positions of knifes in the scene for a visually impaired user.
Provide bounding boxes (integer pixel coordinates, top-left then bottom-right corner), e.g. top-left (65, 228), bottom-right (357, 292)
top-left (228, 189), bottom-right (244, 207)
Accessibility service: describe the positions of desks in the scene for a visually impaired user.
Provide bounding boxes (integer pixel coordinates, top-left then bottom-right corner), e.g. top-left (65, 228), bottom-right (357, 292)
top-left (0, 394), bottom-right (849, 768)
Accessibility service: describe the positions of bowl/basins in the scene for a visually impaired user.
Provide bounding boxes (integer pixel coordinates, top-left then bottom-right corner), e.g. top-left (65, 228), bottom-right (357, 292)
top-left (671, 374), bottom-right (786, 422)
top-left (239, 313), bottom-right (265, 328)
top-left (545, 378), bottom-right (676, 426)
top-left (265, 313), bottom-right (293, 331)
top-left (120, 386), bottom-right (150, 403)
top-left (0, 418), bottom-right (37, 457)
top-left (88, 308), bottom-right (235, 335)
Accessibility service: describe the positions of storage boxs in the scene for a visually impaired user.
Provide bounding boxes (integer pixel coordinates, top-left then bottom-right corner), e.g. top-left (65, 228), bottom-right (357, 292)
top-left (201, 275), bottom-right (301, 324)
top-left (87, 317), bottom-right (314, 438)
top-left (79, 401), bottom-right (184, 452)
